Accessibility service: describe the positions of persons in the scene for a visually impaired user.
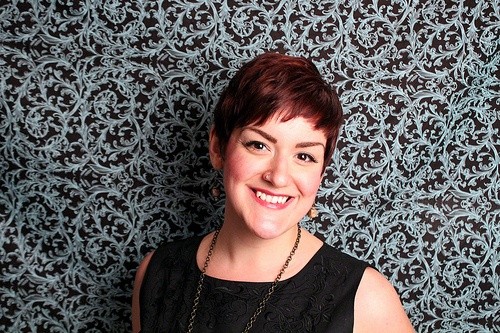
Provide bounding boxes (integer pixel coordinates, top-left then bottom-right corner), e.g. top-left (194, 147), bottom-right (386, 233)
top-left (131, 52), bottom-right (417, 333)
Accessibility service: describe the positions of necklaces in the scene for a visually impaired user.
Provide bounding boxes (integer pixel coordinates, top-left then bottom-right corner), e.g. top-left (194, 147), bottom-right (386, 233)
top-left (186, 218), bottom-right (304, 333)
